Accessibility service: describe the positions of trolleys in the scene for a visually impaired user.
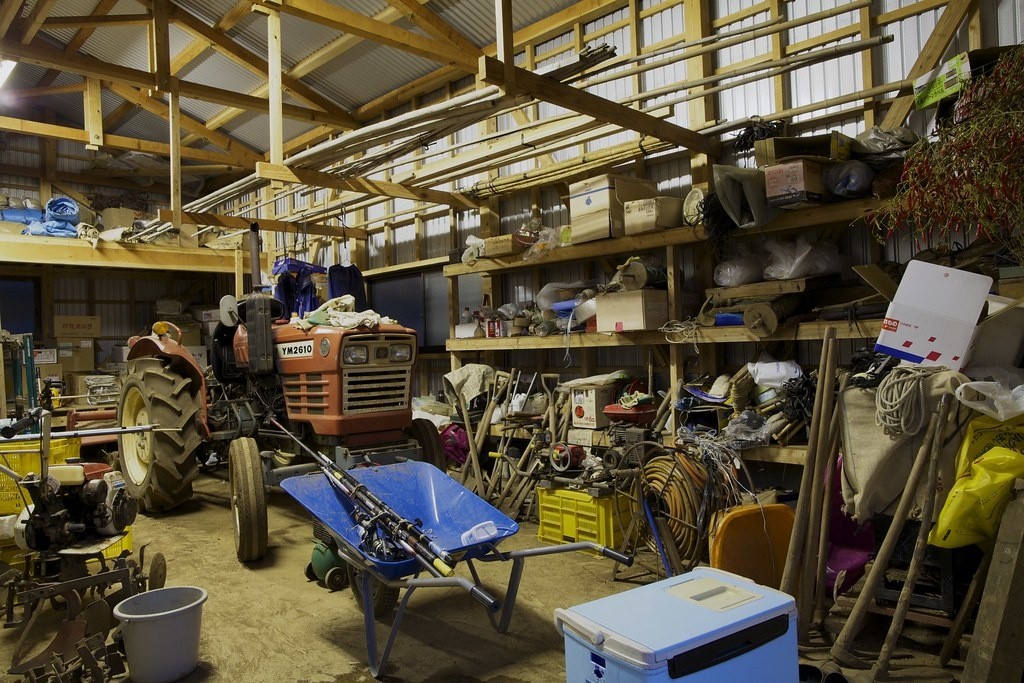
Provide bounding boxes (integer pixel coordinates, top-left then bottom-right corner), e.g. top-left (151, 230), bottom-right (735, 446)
top-left (281, 461), bottom-right (634, 678)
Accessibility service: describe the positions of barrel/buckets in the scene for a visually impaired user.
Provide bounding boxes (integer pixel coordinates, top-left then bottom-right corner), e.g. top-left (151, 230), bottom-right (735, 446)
top-left (111, 585), bottom-right (209, 683)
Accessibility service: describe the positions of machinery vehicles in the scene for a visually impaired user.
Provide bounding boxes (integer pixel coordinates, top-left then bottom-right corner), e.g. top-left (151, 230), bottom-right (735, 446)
top-left (115, 221), bottom-right (447, 563)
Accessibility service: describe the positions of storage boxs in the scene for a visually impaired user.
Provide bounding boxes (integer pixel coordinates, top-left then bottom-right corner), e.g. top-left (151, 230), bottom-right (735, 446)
top-left (102, 208), bottom-right (250, 251)
top-left (553, 567), bottom-right (802, 683)
top-left (754, 131), bottom-right (854, 164)
top-left (623, 196), bottom-right (683, 236)
top-left (483, 130), bottom-right (864, 556)
top-left (913, 43), bottom-right (1022, 111)
top-left (874, 259), bottom-right (1023, 376)
top-left (0, 435), bottom-right (132, 576)
top-left (538, 485), bottom-right (643, 558)
top-left (594, 288), bottom-right (700, 331)
top-left (33, 299), bottom-right (218, 406)
top-left (483, 234), bottom-right (537, 258)
top-left (569, 174), bottom-right (657, 245)
top-left (683, 404), bottom-right (732, 436)
top-left (763, 158), bottom-right (827, 207)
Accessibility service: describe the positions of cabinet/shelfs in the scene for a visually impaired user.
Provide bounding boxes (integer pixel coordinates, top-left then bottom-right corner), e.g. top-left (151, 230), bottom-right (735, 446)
top-left (445, 192), bottom-right (921, 466)
